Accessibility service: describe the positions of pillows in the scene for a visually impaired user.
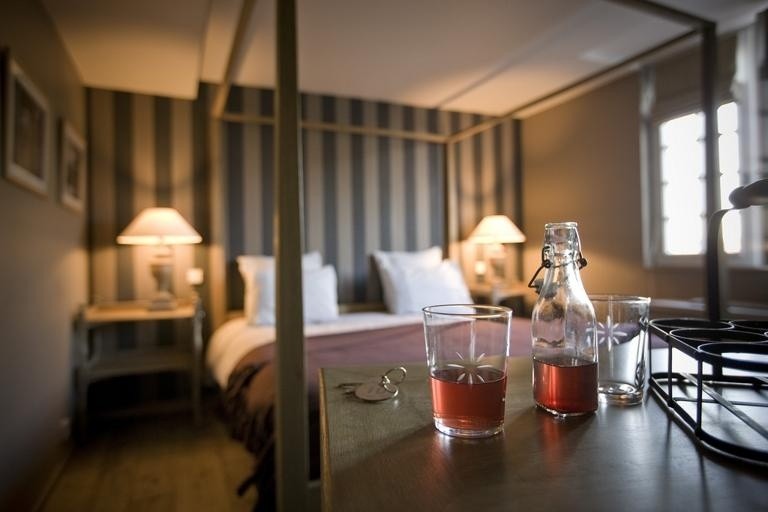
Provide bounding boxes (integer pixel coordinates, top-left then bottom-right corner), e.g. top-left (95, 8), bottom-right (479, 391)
top-left (233, 246), bottom-right (326, 320)
top-left (372, 255), bottom-right (479, 323)
top-left (371, 244), bottom-right (446, 311)
top-left (245, 257), bottom-right (340, 329)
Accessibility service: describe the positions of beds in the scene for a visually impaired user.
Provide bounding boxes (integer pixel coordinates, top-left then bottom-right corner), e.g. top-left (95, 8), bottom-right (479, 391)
top-left (198, 301), bottom-right (681, 512)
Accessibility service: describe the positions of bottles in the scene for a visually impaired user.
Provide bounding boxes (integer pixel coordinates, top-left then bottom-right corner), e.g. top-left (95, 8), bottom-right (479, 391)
top-left (524, 221), bottom-right (600, 420)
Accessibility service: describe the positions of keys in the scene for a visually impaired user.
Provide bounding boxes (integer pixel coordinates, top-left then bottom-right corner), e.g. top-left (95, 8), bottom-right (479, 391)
top-left (338, 366), bottom-right (407, 403)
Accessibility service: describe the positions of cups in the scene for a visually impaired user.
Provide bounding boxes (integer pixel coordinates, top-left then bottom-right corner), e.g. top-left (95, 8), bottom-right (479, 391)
top-left (423, 302), bottom-right (513, 443)
top-left (587, 292), bottom-right (651, 407)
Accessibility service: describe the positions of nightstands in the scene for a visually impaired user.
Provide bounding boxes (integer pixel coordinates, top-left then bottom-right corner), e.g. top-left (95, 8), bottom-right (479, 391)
top-left (65, 294), bottom-right (209, 456)
top-left (463, 273), bottom-right (540, 322)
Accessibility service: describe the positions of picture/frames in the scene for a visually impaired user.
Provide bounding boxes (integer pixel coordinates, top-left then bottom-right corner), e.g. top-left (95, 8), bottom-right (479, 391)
top-left (53, 115), bottom-right (92, 218)
top-left (0, 40), bottom-right (52, 203)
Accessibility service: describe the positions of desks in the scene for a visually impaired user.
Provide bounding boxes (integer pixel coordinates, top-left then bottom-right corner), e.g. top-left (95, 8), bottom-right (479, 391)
top-left (314, 338), bottom-right (767, 512)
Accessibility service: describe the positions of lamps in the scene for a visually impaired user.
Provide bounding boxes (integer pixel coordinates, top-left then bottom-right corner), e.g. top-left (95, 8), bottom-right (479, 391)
top-left (464, 213), bottom-right (529, 289)
top-left (111, 203), bottom-right (206, 313)
